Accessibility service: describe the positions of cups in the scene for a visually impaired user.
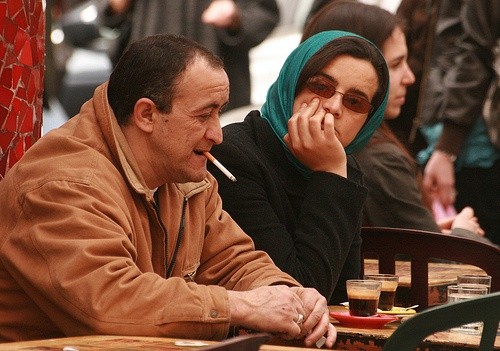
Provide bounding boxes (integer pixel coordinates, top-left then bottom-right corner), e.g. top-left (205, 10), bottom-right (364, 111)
top-left (366, 274), bottom-right (398, 311)
top-left (346, 280), bottom-right (382, 316)
top-left (457, 274), bottom-right (491, 329)
top-left (447, 286), bottom-right (487, 334)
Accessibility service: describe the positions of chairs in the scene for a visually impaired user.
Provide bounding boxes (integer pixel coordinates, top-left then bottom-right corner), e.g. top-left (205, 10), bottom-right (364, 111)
top-left (361, 226), bottom-right (500, 314)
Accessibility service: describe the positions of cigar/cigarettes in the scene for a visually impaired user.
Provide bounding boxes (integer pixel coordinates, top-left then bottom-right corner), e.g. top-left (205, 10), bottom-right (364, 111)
top-left (202, 151), bottom-right (236, 181)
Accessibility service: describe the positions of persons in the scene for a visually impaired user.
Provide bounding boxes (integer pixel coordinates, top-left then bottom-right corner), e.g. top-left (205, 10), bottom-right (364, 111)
top-left (0, 0), bottom-right (500, 350)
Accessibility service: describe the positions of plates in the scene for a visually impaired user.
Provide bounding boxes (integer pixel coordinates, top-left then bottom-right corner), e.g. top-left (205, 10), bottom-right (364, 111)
top-left (328, 312), bottom-right (397, 328)
top-left (342, 301), bottom-right (417, 315)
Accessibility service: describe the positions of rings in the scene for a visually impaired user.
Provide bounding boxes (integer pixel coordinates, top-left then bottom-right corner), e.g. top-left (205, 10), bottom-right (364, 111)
top-left (297, 312), bottom-right (304, 326)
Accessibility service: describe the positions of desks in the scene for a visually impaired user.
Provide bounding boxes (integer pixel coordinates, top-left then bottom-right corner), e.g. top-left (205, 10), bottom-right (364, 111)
top-left (236, 305), bottom-right (500, 351)
top-left (362, 258), bottom-right (491, 308)
top-left (0, 334), bottom-right (272, 351)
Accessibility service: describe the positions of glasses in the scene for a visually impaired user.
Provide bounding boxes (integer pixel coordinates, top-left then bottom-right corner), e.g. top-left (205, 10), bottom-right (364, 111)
top-left (305, 75), bottom-right (374, 115)
